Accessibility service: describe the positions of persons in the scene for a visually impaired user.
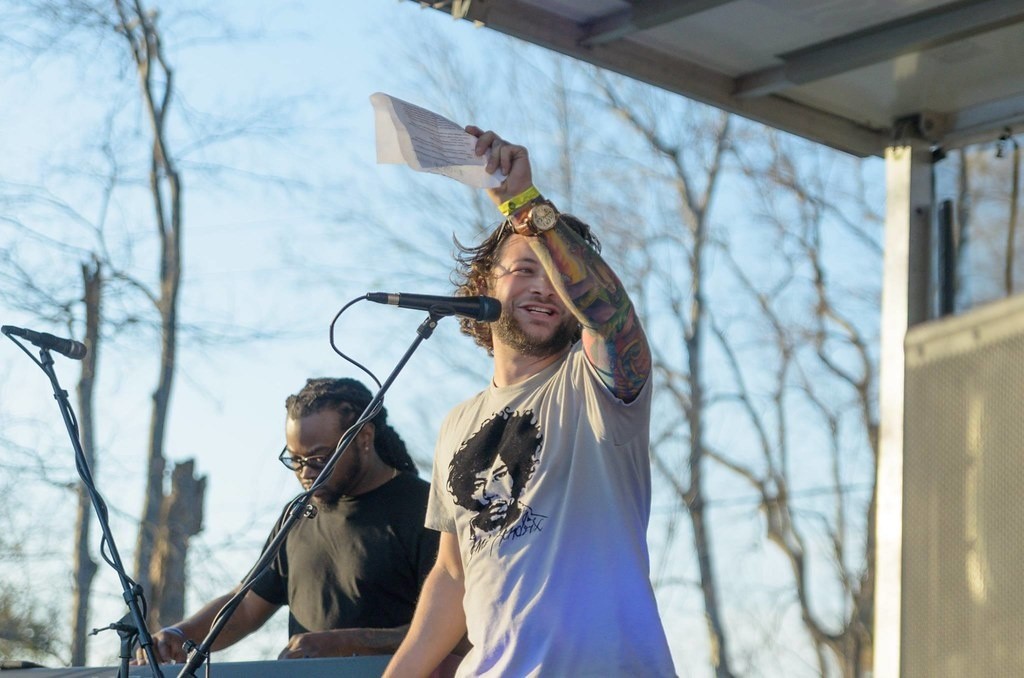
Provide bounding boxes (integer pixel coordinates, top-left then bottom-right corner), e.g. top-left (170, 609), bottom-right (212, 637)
top-left (127, 378), bottom-right (443, 666)
top-left (379, 125), bottom-right (683, 678)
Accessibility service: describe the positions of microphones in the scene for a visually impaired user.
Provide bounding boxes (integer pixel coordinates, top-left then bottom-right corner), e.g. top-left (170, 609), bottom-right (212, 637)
top-left (0, 325), bottom-right (88, 361)
top-left (366, 292), bottom-right (502, 324)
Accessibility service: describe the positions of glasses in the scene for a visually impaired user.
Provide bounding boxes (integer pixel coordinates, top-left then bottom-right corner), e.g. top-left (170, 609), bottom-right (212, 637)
top-left (279, 444), bottom-right (339, 471)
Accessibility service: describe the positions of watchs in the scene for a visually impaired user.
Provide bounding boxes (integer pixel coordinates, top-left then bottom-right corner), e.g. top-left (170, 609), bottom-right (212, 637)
top-left (511, 198), bottom-right (560, 237)
